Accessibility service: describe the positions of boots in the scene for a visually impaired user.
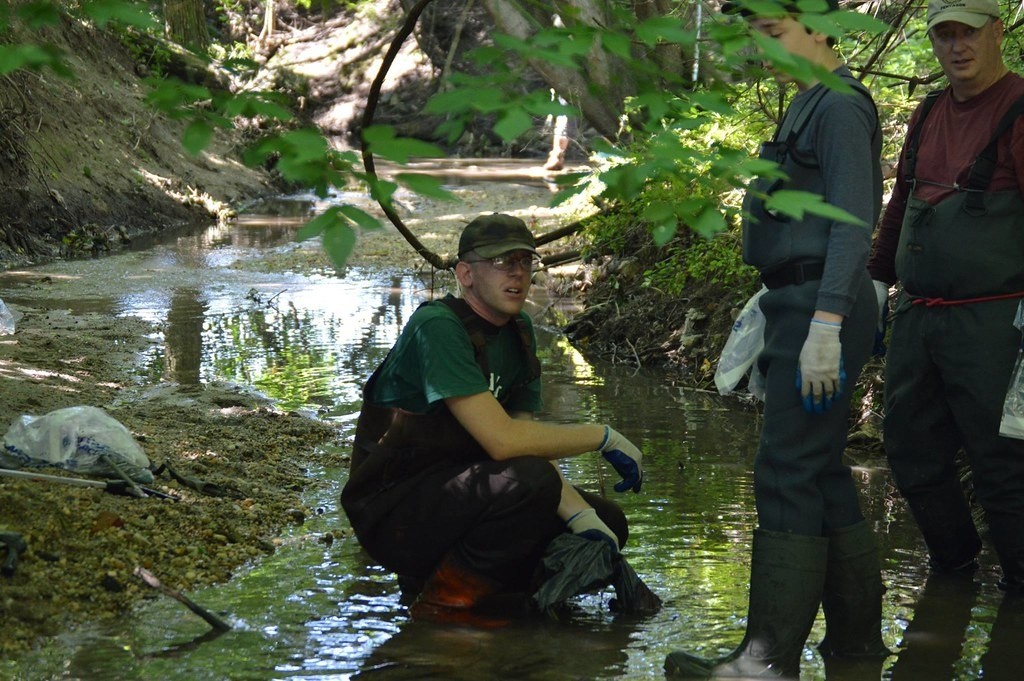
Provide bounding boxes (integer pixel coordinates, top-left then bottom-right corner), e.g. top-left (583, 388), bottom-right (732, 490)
top-left (664, 527), bottom-right (830, 681)
top-left (816, 528), bottom-right (893, 655)
top-left (907, 467), bottom-right (982, 565)
top-left (990, 521), bottom-right (1024, 588)
top-left (407, 561), bottom-right (511, 629)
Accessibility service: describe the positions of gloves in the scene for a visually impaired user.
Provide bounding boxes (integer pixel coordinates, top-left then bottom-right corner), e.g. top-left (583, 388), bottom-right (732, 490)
top-left (872, 279), bottom-right (891, 357)
top-left (795, 319), bottom-right (847, 416)
top-left (564, 509), bottom-right (619, 556)
top-left (595, 425), bottom-right (643, 493)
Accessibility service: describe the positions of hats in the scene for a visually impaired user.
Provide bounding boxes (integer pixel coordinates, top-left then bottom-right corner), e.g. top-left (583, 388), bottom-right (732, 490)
top-left (721, 0), bottom-right (839, 21)
top-left (924, 0), bottom-right (1000, 38)
top-left (458, 212), bottom-right (541, 260)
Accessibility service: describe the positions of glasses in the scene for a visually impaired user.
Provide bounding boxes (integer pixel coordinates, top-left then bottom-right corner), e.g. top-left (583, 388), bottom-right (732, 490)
top-left (466, 255), bottom-right (539, 272)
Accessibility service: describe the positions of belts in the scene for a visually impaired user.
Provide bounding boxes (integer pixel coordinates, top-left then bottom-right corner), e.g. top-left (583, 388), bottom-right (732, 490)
top-left (761, 262), bottom-right (824, 290)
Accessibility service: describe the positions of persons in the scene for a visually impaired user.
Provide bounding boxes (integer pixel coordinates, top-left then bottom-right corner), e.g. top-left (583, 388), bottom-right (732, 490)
top-left (339, 215), bottom-right (643, 625)
top-left (662, 0), bottom-right (1024, 681)
